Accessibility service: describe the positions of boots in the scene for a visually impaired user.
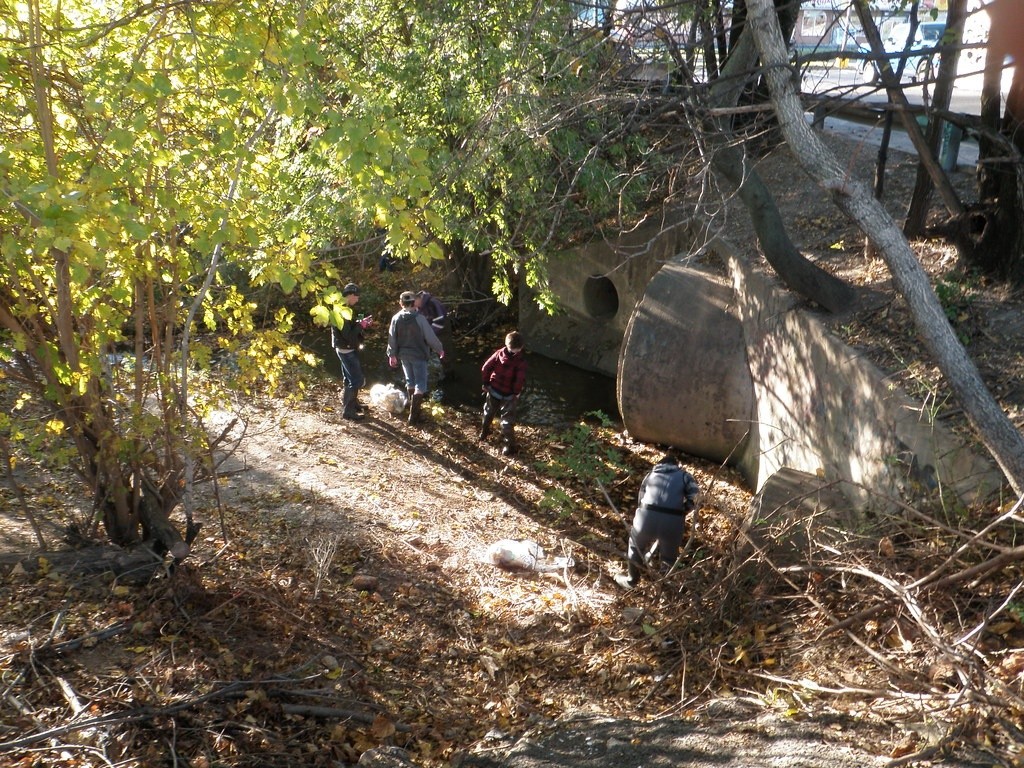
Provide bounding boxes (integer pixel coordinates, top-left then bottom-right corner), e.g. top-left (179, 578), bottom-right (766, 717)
top-left (407, 388), bottom-right (414, 399)
top-left (407, 394), bottom-right (426, 424)
top-left (478, 415), bottom-right (493, 440)
top-left (503, 429), bottom-right (521, 456)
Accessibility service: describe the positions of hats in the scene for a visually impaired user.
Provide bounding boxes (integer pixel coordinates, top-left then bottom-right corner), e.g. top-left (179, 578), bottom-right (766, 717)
top-left (400, 291), bottom-right (416, 302)
top-left (343, 282), bottom-right (361, 295)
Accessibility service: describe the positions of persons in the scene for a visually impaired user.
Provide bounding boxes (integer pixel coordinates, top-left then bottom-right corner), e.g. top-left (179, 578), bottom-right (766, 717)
top-left (479, 331), bottom-right (526, 455)
top-left (409, 292), bottom-right (455, 386)
top-left (386, 291), bottom-right (446, 425)
top-left (628, 456), bottom-right (699, 588)
top-left (331, 283), bottom-right (373, 421)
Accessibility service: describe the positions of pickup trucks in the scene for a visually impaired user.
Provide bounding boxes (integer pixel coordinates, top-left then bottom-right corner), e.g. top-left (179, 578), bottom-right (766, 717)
top-left (857, 24), bottom-right (946, 87)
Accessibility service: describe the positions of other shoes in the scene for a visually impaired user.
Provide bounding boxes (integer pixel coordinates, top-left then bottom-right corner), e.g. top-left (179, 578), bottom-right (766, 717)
top-left (356, 404), bottom-right (368, 411)
top-left (343, 412), bottom-right (365, 421)
